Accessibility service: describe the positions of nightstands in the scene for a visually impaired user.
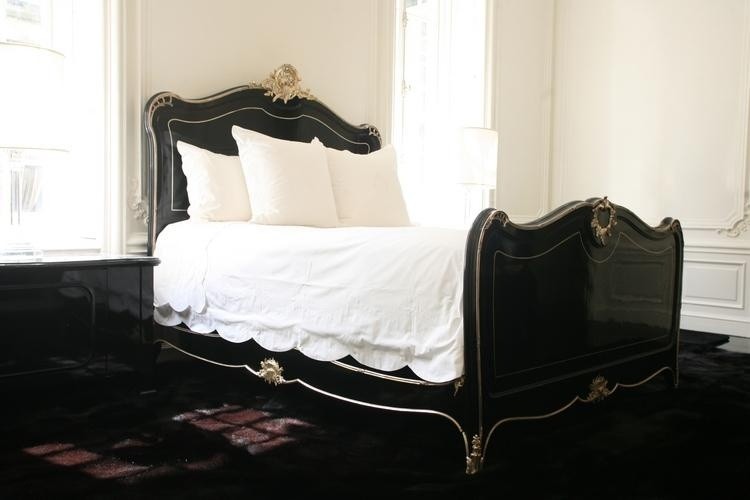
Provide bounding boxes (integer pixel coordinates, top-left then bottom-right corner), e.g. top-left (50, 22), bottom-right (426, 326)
top-left (1, 248), bottom-right (168, 401)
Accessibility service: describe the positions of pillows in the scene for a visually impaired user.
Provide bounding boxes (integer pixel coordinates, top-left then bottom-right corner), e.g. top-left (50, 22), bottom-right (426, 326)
top-left (173, 134), bottom-right (264, 231)
top-left (227, 119), bottom-right (340, 229)
top-left (325, 144), bottom-right (416, 230)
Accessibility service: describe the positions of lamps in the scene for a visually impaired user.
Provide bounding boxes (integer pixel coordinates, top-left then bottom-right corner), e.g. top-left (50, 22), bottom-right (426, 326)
top-left (4, 37), bottom-right (75, 262)
top-left (447, 127), bottom-right (497, 222)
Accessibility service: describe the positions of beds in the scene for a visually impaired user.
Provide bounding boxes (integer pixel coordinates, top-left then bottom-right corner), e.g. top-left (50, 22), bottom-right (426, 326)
top-left (140, 62), bottom-right (690, 481)
